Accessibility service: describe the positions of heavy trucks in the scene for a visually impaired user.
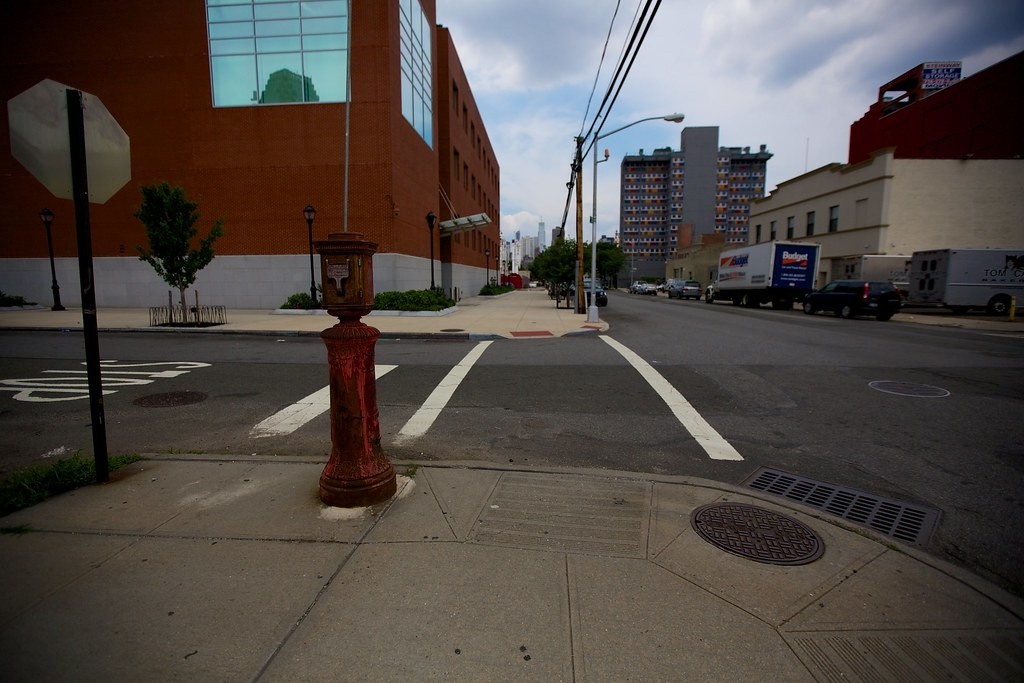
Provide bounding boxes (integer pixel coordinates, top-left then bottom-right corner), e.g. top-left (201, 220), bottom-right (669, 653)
top-left (705, 240), bottom-right (822, 310)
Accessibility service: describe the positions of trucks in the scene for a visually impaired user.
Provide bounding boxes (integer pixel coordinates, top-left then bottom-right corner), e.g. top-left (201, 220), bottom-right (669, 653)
top-left (910, 248), bottom-right (1024, 318)
top-left (861, 254), bottom-right (913, 308)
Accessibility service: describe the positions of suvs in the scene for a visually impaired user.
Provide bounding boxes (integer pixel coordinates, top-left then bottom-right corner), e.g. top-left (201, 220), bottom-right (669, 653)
top-left (803, 280), bottom-right (905, 321)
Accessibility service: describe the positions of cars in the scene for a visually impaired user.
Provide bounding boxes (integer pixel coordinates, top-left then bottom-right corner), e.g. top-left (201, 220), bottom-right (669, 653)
top-left (668, 280), bottom-right (702, 300)
top-left (561, 282), bottom-right (576, 296)
top-left (584, 279), bottom-right (608, 307)
top-left (630, 279), bottom-right (675, 296)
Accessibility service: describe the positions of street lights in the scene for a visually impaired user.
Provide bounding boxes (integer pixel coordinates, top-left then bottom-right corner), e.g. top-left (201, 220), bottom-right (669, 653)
top-left (425, 211), bottom-right (437, 290)
top-left (507, 263), bottom-right (511, 286)
top-left (588, 114), bottom-right (685, 323)
top-left (39, 208), bottom-right (66, 312)
top-left (502, 260), bottom-right (506, 285)
top-left (303, 205), bottom-right (317, 300)
top-left (495, 255), bottom-right (499, 285)
top-left (485, 248), bottom-right (490, 285)
